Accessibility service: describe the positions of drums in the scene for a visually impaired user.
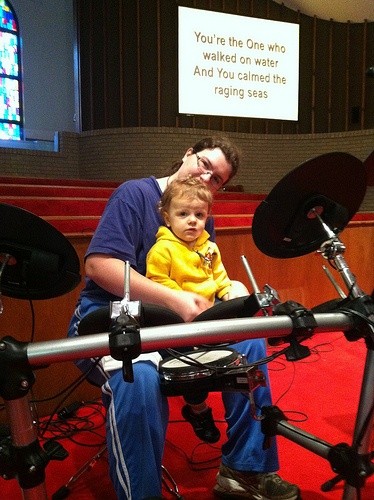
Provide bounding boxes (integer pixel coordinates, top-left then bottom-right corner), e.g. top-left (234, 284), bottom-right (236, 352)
top-left (156, 346), bottom-right (243, 384)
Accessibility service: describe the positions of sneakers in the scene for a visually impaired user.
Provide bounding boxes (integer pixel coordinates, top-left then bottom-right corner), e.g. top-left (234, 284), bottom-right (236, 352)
top-left (214, 463), bottom-right (299, 500)
top-left (181, 404), bottom-right (221, 443)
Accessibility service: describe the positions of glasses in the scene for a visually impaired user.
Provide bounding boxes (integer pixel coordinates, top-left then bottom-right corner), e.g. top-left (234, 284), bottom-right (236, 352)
top-left (195, 152), bottom-right (226, 192)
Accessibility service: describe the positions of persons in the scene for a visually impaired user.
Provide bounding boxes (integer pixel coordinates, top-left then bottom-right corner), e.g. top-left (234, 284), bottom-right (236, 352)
top-left (69, 135), bottom-right (302, 500)
top-left (146, 173), bottom-right (234, 443)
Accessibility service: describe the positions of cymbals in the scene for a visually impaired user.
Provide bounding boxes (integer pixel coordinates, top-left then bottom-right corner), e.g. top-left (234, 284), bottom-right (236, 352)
top-left (250, 151), bottom-right (368, 259)
top-left (78, 304), bottom-right (186, 337)
top-left (0, 202), bottom-right (82, 300)
top-left (192, 295), bottom-right (262, 323)
